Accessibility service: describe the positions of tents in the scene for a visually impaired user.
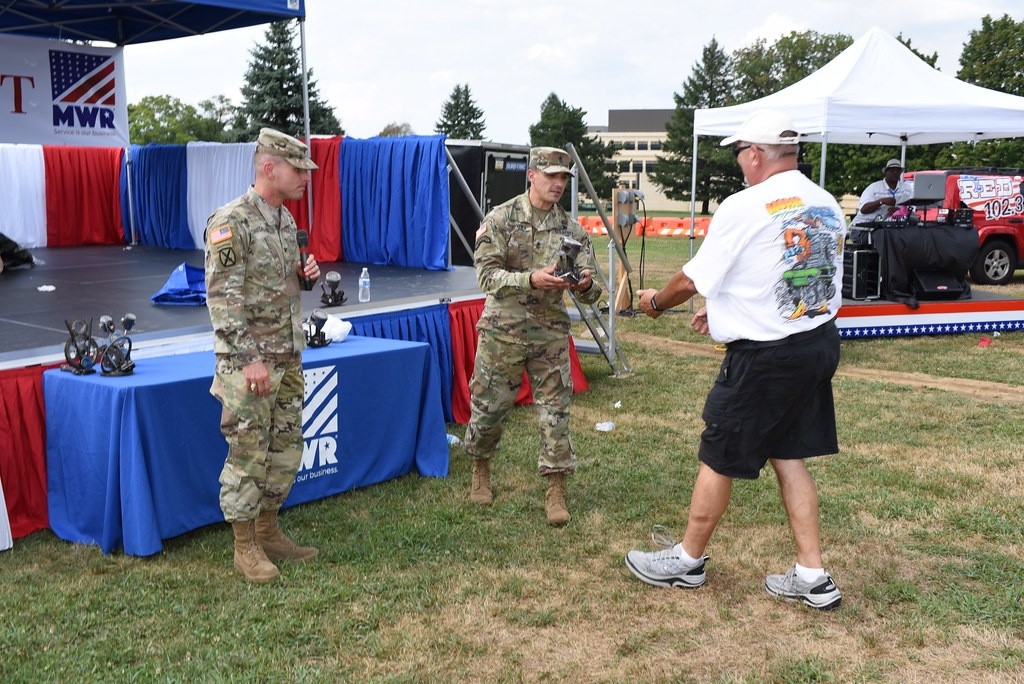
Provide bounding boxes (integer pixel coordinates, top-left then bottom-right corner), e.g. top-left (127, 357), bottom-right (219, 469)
top-left (0, 0), bottom-right (313, 235)
top-left (688, 25), bottom-right (1024, 313)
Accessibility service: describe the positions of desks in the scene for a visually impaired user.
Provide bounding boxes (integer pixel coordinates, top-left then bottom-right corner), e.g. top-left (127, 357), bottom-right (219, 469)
top-left (43, 334), bottom-right (449, 557)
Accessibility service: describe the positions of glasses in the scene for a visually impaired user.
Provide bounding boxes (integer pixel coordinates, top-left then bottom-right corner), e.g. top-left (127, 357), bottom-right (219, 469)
top-left (733, 145), bottom-right (764, 158)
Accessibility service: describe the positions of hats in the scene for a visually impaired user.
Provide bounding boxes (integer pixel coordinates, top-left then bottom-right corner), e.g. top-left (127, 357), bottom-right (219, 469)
top-left (720, 109), bottom-right (800, 147)
top-left (255, 127), bottom-right (319, 170)
top-left (881, 159), bottom-right (901, 174)
top-left (529, 147), bottom-right (575, 177)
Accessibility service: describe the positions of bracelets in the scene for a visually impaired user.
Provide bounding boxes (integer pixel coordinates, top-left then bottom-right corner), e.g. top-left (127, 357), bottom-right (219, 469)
top-left (880, 199), bottom-right (883, 205)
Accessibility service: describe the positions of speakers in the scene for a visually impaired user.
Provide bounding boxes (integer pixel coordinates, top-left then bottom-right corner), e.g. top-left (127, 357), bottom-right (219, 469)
top-left (908, 270), bottom-right (964, 301)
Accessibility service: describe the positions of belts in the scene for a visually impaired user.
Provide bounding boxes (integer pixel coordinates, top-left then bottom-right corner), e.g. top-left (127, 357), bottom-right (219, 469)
top-left (726, 317), bottom-right (835, 352)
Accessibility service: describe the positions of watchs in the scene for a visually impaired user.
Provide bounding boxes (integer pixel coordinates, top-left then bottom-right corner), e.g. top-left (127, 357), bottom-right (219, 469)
top-left (650, 292), bottom-right (665, 312)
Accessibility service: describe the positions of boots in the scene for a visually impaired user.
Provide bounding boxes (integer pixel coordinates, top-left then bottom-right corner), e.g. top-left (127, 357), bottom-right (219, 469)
top-left (544, 472), bottom-right (571, 526)
top-left (255, 509), bottom-right (319, 562)
top-left (471, 460), bottom-right (492, 505)
top-left (230, 520), bottom-right (280, 584)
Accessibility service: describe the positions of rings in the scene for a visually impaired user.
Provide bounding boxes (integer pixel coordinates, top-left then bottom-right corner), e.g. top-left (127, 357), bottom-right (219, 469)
top-left (251, 383), bottom-right (257, 392)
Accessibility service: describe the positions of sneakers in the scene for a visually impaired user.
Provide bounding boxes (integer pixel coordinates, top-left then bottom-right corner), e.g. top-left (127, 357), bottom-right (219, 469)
top-left (624, 543), bottom-right (710, 590)
top-left (765, 565), bottom-right (842, 611)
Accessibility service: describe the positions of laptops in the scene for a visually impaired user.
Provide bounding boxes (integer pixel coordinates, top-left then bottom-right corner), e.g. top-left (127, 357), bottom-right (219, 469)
top-left (897, 173), bottom-right (947, 206)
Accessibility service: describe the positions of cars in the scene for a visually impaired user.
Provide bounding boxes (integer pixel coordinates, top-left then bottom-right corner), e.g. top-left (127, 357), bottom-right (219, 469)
top-left (895, 168), bottom-right (1023, 285)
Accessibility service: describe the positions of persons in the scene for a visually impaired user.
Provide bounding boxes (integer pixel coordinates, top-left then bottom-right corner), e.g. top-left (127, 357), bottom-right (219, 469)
top-left (847, 159), bottom-right (913, 244)
top-left (624, 109), bottom-right (842, 610)
top-left (465, 147), bottom-right (601, 524)
top-left (203, 127), bottom-right (321, 583)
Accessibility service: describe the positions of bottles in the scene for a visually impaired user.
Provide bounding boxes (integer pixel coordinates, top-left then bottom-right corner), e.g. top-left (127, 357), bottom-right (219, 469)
top-left (595, 422), bottom-right (616, 432)
top-left (359, 267), bottom-right (370, 302)
top-left (446, 433), bottom-right (464, 448)
top-left (616, 369), bottom-right (635, 380)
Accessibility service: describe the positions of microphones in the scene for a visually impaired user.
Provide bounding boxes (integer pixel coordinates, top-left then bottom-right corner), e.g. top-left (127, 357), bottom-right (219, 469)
top-left (295, 229), bottom-right (313, 290)
